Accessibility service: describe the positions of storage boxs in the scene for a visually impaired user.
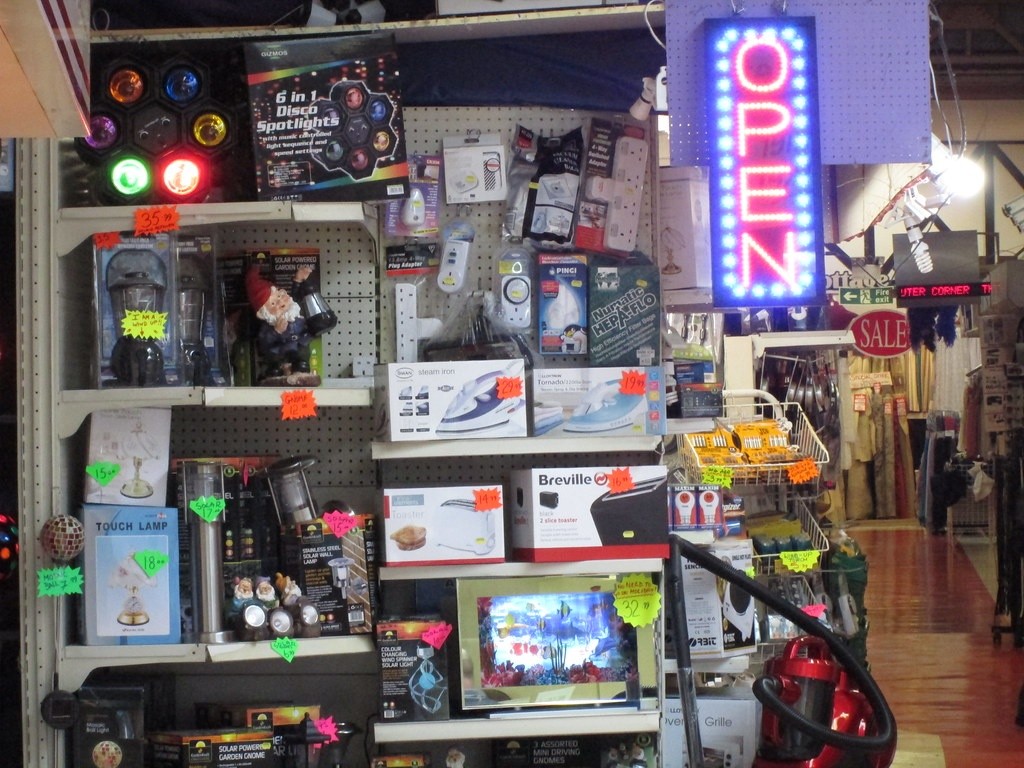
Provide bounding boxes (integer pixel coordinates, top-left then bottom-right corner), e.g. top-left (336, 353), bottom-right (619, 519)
top-left (662, 687), bottom-right (763, 768)
top-left (218, 31), bottom-right (413, 203)
top-left (587, 249), bottom-right (660, 367)
top-left (71, 501), bottom-right (182, 646)
top-left (147, 729), bottom-right (274, 768)
top-left (441, 573), bottom-right (660, 712)
top-left (91, 230), bottom-right (182, 387)
top-left (759, 614), bottom-right (799, 654)
top-left (532, 249), bottom-right (588, 355)
top-left (373, 358), bottom-right (529, 442)
top-left (370, 484), bottom-right (506, 567)
top-left (377, 618), bottom-right (450, 723)
top-left (371, 751), bottom-right (433, 768)
top-left (527, 341), bottom-right (721, 436)
top-left (220, 703), bottom-right (322, 768)
top-left (223, 246), bottom-right (324, 386)
top-left (84, 405), bottom-right (174, 506)
top-left (511, 464), bottom-right (672, 562)
top-left (279, 513), bottom-right (382, 635)
top-left (599, 732), bottom-right (658, 768)
top-left (672, 483), bottom-right (748, 538)
top-left (679, 538), bottom-right (758, 662)
top-left (70, 679), bottom-right (154, 768)
top-left (491, 738), bottom-right (529, 768)
top-left (175, 234), bottom-right (221, 388)
top-left (529, 734), bottom-right (602, 768)
top-left (195, 699), bottom-right (295, 732)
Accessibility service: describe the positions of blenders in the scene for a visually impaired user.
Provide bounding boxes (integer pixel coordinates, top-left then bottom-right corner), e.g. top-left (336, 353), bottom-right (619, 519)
top-left (105, 247), bottom-right (170, 389)
top-left (174, 254), bottom-right (221, 386)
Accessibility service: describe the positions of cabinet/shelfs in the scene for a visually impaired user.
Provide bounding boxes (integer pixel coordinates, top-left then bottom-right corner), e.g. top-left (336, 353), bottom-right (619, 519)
top-left (12, 6), bottom-right (858, 768)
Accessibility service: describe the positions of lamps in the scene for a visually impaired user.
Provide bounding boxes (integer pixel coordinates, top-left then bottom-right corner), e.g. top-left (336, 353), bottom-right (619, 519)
top-left (930, 132), bottom-right (986, 200)
top-left (905, 214), bottom-right (934, 274)
